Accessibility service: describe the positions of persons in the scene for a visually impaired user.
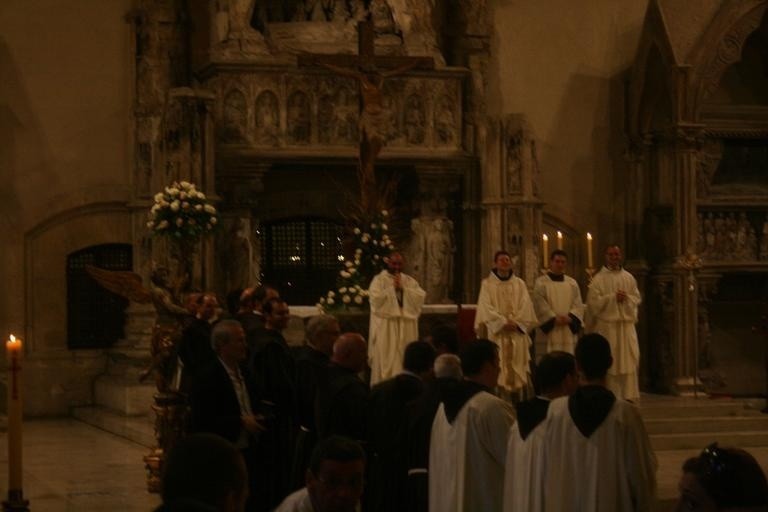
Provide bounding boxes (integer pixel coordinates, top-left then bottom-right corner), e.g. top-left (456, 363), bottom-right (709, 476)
top-left (532, 250), bottom-right (585, 355)
top-left (472, 251), bottom-right (534, 407)
top-left (152, 270), bottom-right (192, 392)
top-left (587, 247), bottom-right (642, 402)
top-left (676, 441), bottom-right (768, 511)
top-left (220, 88), bottom-right (459, 144)
top-left (696, 206), bottom-right (767, 260)
top-left (155, 282), bottom-right (658, 510)
top-left (367, 253), bottom-right (427, 386)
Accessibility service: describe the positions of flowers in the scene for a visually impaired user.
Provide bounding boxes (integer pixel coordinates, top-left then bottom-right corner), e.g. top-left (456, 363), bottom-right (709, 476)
top-left (315, 210), bottom-right (396, 314)
top-left (147, 182), bottom-right (217, 234)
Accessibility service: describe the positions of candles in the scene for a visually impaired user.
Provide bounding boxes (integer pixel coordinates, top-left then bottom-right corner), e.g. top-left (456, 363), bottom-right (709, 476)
top-left (542, 233), bottom-right (549, 268)
top-left (586, 232), bottom-right (593, 268)
top-left (6, 333), bottom-right (24, 490)
top-left (556, 231), bottom-right (563, 250)
